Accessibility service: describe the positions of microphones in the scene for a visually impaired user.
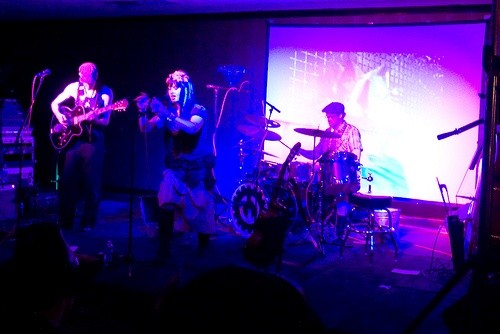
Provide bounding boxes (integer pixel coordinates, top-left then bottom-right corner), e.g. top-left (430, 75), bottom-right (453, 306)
top-left (265, 101), bottom-right (281, 113)
top-left (36, 69), bottom-right (51, 77)
top-left (207, 85), bottom-right (223, 89)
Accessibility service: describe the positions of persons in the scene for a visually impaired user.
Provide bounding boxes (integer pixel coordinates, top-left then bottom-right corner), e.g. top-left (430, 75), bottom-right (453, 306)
top-left (0, 221), bottom-right (74, 334)
top-left (290, 101), bottom-right (363, 191)
top-left (158, 266), bottom-right (329, 334)
top-left (51, 62), bottom-right (113, 239)
top-left (134, 70), bottom-right (216, 257)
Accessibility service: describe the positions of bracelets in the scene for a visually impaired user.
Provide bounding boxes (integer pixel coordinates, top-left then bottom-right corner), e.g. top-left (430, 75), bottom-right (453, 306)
top-left (138, 112), bottom-right (145, 117)
top-left (167, 113), bottom-right (175, 121)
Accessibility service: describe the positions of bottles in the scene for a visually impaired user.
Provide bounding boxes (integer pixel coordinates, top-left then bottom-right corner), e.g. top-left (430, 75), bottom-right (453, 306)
top-left (103, 241), bottom-right (114, 269)
top-left (68, 246), bottom-right (81, 274)
top-left (120, 246), bottom-right (138, 278)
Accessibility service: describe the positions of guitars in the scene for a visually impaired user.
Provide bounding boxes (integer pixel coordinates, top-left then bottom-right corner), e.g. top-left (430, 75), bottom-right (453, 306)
top-left (243, 141), bottom-right (302, 269)
top-left (49, 97), bottom-right (130, 150)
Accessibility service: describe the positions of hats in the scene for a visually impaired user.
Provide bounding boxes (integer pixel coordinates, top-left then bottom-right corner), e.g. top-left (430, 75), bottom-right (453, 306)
top-left (78, 62), bottom-right (97, 83)
top-left (322, 102), bottom-right (344, 114)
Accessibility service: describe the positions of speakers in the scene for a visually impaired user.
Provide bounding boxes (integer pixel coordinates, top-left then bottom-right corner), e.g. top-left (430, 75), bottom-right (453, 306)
top-left (177, 264), bottom-right (329, 334)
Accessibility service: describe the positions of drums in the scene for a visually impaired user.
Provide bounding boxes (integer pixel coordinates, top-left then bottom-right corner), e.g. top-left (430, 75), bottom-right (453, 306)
top-left (318, 150), bottom-right (361, 195)
top-left (228, 178), bottom-right (299, 242)
top-left (287, 161), bottom-right (313, 185)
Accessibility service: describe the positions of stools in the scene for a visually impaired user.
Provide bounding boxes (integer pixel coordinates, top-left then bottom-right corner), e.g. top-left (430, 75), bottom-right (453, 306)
top-left (339, 190), bottom-right (398, 265)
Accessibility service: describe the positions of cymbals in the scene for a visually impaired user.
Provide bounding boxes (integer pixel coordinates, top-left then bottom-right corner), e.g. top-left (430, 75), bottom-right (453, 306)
top-left (239, 126), bottom-right (282, 141)
top-left (293, 128), bottom-right (342, 138)
top-left (245, 114), bottom-right (281, 128)
